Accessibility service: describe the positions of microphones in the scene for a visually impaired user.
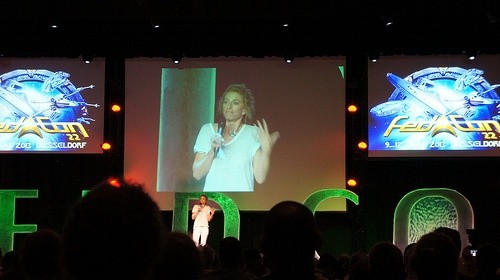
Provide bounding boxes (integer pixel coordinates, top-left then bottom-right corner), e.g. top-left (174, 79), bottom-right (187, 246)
top-left (199, 206), bottom-right (203, 212)
top-left (213, 119), bottom-right (227, 154)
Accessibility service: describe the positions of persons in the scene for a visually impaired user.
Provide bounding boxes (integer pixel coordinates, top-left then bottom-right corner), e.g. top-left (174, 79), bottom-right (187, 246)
top-left (0, 181), bottom-right (499, 280)
top-left (191, 84), bottom-right (281, 193)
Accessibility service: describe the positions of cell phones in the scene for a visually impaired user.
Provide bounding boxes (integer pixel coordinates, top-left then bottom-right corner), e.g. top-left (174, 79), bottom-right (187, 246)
top-left (469, 247), bottom-right (478, 257)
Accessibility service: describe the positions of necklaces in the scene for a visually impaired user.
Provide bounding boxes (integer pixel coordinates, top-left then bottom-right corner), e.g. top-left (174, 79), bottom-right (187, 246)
top-left (224, 127), bottom-right (240, 137)
